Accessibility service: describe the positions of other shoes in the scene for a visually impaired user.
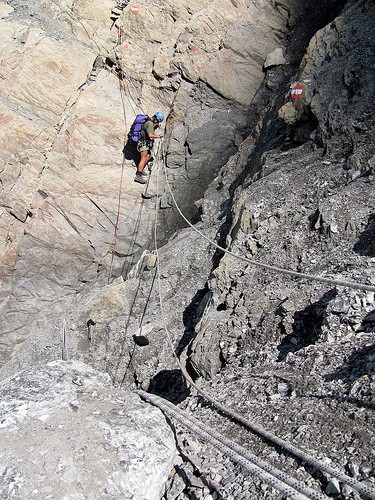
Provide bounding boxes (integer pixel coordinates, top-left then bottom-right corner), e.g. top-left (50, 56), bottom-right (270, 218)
top-left (142, 171), bottom-right (147, 176)
top-left (134, 175), bottom-right (147, 184)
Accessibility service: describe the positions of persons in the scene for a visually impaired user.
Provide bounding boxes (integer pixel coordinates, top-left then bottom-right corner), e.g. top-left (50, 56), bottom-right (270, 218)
top-left (133, 111), bottom-right (163, 184)
top-left (278, 71), bottom-right (303, 144)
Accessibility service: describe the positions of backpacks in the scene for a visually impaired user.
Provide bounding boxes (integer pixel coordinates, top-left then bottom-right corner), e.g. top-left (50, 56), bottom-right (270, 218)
top-left (128, 113), bottom-right (152, 142)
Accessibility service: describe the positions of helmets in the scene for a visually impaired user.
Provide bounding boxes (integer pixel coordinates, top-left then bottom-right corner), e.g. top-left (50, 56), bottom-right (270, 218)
top-left (154, 111), bottom-right (164, 122)
